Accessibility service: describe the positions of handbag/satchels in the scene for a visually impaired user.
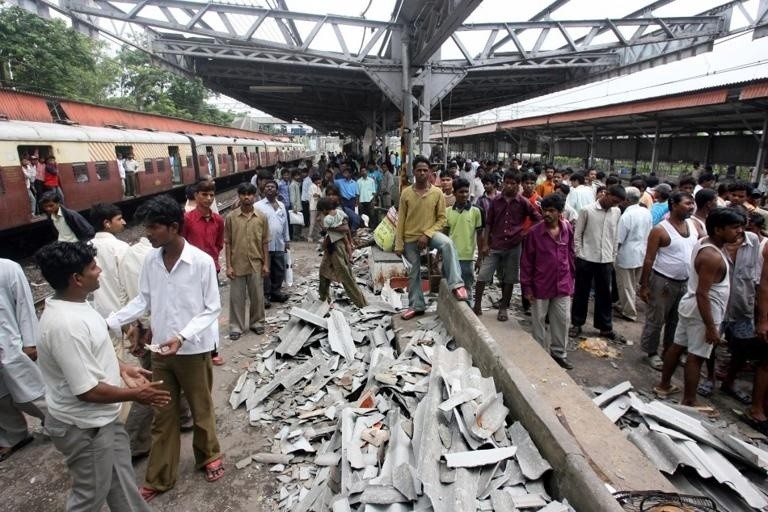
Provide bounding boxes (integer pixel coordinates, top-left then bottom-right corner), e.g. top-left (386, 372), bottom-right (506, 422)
top-left (289, 210), bottom-right (305, 226)
top-left (284, 249), bottom-right (294, 288)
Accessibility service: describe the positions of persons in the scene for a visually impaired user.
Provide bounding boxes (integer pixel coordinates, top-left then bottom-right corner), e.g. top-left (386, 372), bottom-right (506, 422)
top-left (394, 155), bottom-right (468, 320)
top-left (19, 150), bottom-right (65, 219)
top-left (428, 150), bottom-right (768, 430)
top-left (315, 194), bottom-right (368, 309)
top-left (251, 151), bottom-right (400, 245)
top-left (116, 152), bottom-right (139, 197)
top-left (0, 173), bottom-right (290, 511)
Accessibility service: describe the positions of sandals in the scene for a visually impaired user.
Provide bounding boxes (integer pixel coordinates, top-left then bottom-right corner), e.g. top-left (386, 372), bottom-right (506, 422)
top-left (470, 299), bottom-right (534, 321)
top-left (264, 299), bottom-right (271, 310)
top-left (273, 293), bottom-right (288, 301)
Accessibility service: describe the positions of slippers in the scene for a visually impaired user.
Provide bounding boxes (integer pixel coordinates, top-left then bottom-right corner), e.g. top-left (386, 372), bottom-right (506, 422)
top-left (213, 355), bottom-right (224, 367)
top-left (602, 330), bottom-right (627, 345)
top-left (615, 312), bottom-right (632, 321)
top-left (138, 486), bottom-right (158, 502)
top-left (646, 349), bottom-right (753, 419)
top-left (1, 435), bottom-right (35, 462)
top-left (253, 327), bottom-right (266, 336)
top-left (569, 326), bottom-right (582, 338)
top-left (231, 331), bottom-right (241, 340)
top-left (739, 409), bottom-right (767, 436)
top-left (204, 459), bottom-right (224, 482)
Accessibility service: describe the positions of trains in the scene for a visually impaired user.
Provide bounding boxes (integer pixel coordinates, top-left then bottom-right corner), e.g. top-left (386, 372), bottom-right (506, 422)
top-left (0, 116), bottom-right (315, 236)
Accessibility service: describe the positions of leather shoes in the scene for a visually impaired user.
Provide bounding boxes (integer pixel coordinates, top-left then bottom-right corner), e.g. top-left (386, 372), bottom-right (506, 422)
top-left (552, 353), bottom-right (573, 369)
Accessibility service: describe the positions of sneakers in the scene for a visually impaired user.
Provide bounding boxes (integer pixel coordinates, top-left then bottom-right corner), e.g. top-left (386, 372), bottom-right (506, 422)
top-left (401, 309), bottom-right (425, 319)
top-left (456, 288), bottom-right (470, 297)
